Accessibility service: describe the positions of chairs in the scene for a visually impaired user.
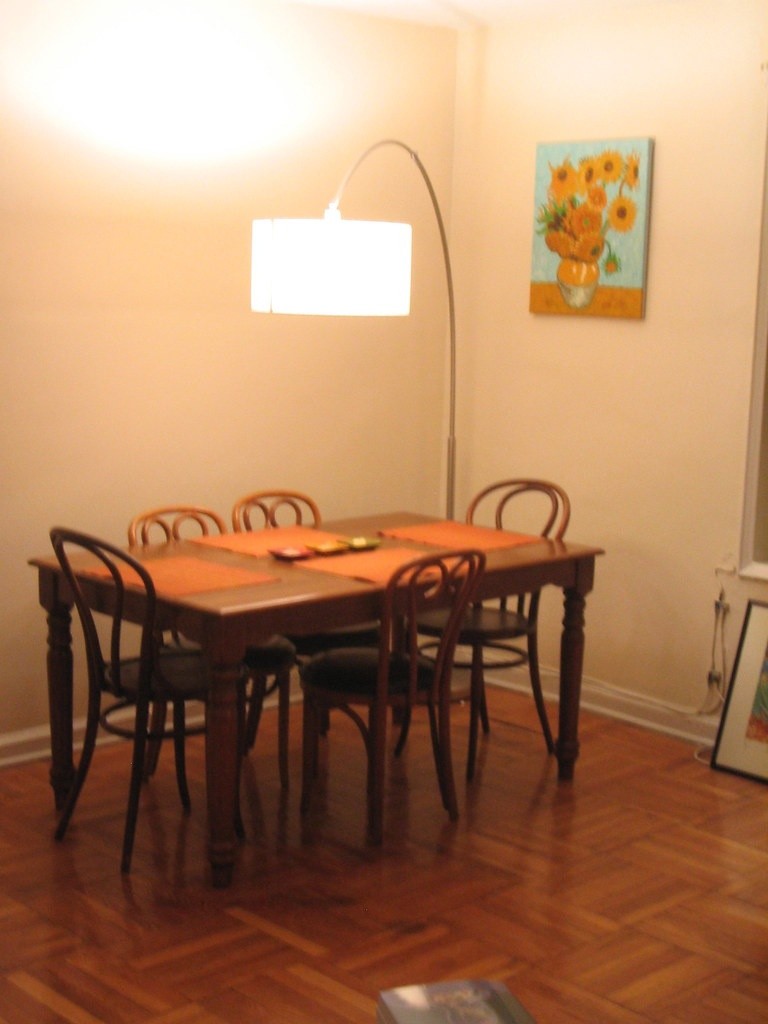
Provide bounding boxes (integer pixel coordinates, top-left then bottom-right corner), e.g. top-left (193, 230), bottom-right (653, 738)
top-left (49, 526), bottom-right (247, 878)
top-left (128, 507), bottom-right (290, 788)
top-left (304, 550), bottom-right (486, 843)
top-left (232, 489), bottom-right (366, 734)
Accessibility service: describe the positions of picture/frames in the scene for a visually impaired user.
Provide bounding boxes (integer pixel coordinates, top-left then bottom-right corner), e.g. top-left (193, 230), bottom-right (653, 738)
top-left (709, 600), bottom-right (768, 786)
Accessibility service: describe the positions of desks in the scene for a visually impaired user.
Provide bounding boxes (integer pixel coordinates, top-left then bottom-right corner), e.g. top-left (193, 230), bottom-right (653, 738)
top-left (27, 510), bottom-right (606, 887)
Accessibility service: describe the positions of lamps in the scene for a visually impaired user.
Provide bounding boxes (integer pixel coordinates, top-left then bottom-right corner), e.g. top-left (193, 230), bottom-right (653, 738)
top-left (251, 139), bottom-right (472, 705)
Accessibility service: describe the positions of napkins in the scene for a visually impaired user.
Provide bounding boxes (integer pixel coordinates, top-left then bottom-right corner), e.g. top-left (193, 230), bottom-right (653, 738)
top-left (186, 525), bottom-right (354, 557)
top-left (294, 547), bottom-right (480, 586)
top-left (80, 554), bottom-right (279, 597)
top-left (394, 478), bottom-right (571, 790)
top-left (377, 520), bottom-right (547, 552)
